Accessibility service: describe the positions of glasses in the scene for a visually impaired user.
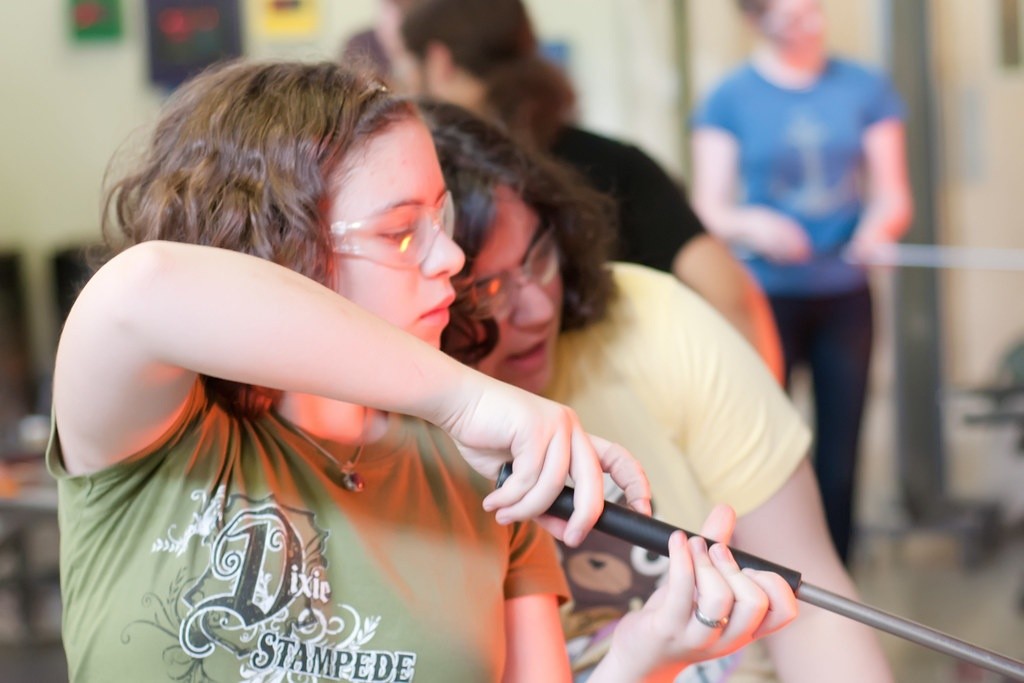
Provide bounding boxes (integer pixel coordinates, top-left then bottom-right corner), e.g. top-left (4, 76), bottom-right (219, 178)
top-left (322, 187), bottom-right (455, 269)
top-left (451, 223), bottom-right (559, 322)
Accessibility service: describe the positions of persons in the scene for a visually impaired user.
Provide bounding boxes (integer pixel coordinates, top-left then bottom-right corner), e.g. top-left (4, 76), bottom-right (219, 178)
top-left (372, 0), bottom-right (786, 392)
top-left (341, 30), bottom-right (392, 93)
top-left (390, 99), bottom-right (892, 683)
top-left (44, 53), bottom-right (796, 682)
top-left (686, 0), bottom-right (914, 581)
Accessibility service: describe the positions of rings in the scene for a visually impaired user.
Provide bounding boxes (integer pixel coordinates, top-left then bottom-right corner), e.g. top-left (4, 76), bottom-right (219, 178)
top-left (695, 604), bottom-right (731, 629)
